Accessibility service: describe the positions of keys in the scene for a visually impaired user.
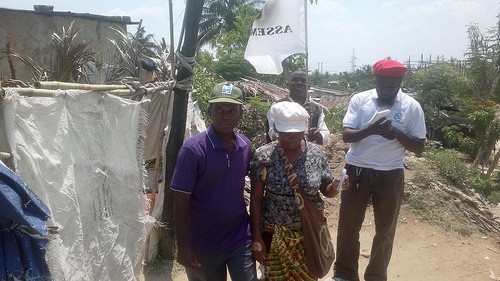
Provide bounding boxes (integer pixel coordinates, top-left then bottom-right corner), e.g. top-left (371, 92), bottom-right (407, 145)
top-left (354, 167), bottom-right (362, 189)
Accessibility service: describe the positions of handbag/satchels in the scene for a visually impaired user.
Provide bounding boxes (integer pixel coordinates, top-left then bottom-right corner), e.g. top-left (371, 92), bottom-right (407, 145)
top-left (277, 141), bottom-right (335, 279)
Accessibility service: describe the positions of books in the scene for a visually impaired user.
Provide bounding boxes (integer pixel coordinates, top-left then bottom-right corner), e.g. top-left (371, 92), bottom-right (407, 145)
top-left (368, 109), bottom-right (391, 125)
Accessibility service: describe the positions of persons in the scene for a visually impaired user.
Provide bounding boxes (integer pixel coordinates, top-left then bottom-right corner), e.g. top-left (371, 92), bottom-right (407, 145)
top-left (250, 101), bottom-right (350, 281)
top-left (266, 70), bottom-right (330, 147)
top-left (333, 60), bottom-right (426, 281)
top-left (170, 80), bottom-right (257, 281)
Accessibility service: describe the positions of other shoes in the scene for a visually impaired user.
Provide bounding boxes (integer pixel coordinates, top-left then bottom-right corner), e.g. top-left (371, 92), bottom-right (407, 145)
top-left (256, 264), bottom-right (265, 280)
top-left (325, 275), bottom-right (350, 281)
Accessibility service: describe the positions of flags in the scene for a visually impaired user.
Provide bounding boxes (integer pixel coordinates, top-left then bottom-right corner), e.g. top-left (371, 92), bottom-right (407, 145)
top-left (244, 0), bottom-right (308, 75)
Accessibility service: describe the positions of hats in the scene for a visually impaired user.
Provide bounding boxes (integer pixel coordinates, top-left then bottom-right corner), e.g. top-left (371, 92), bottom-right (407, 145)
top-left (208, 81), bottom-right (244, 105)
top-left (270, 101), bottom-right (310, 132)
top-left (373, 59), bottom-right (408, 77)
